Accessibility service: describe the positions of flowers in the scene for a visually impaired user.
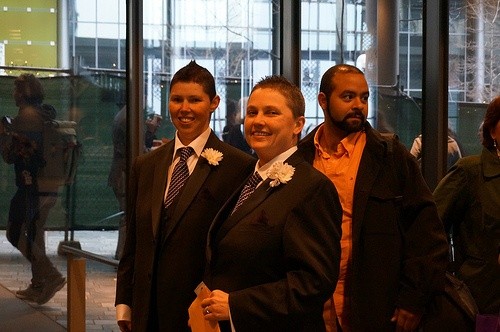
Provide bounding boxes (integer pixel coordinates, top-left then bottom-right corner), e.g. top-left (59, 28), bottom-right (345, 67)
top-left (200, 147), bottom-right (223, 167)
top-left (264, 162), bottom-right (295, 192)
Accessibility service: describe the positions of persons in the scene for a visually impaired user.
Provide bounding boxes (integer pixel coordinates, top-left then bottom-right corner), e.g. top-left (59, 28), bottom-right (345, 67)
top-left (187, 74), bottom-right (342, 332)
top-left (299, 64), bottom-right (449, 332)
top-left (214, 97), bottom-right (259, 159)
top-left (432, 95), bottom-right (500, 332)
top-left (108, 94), bottom-right (162, 269)
top-left (114, 60), bottom-right (258, 332)
top-left (0, 73), bottom-right (66, 305)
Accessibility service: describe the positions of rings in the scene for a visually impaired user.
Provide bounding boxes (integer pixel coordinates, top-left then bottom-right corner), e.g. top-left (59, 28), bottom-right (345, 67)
top-left (206, 305), bottom-right (210, 314)
